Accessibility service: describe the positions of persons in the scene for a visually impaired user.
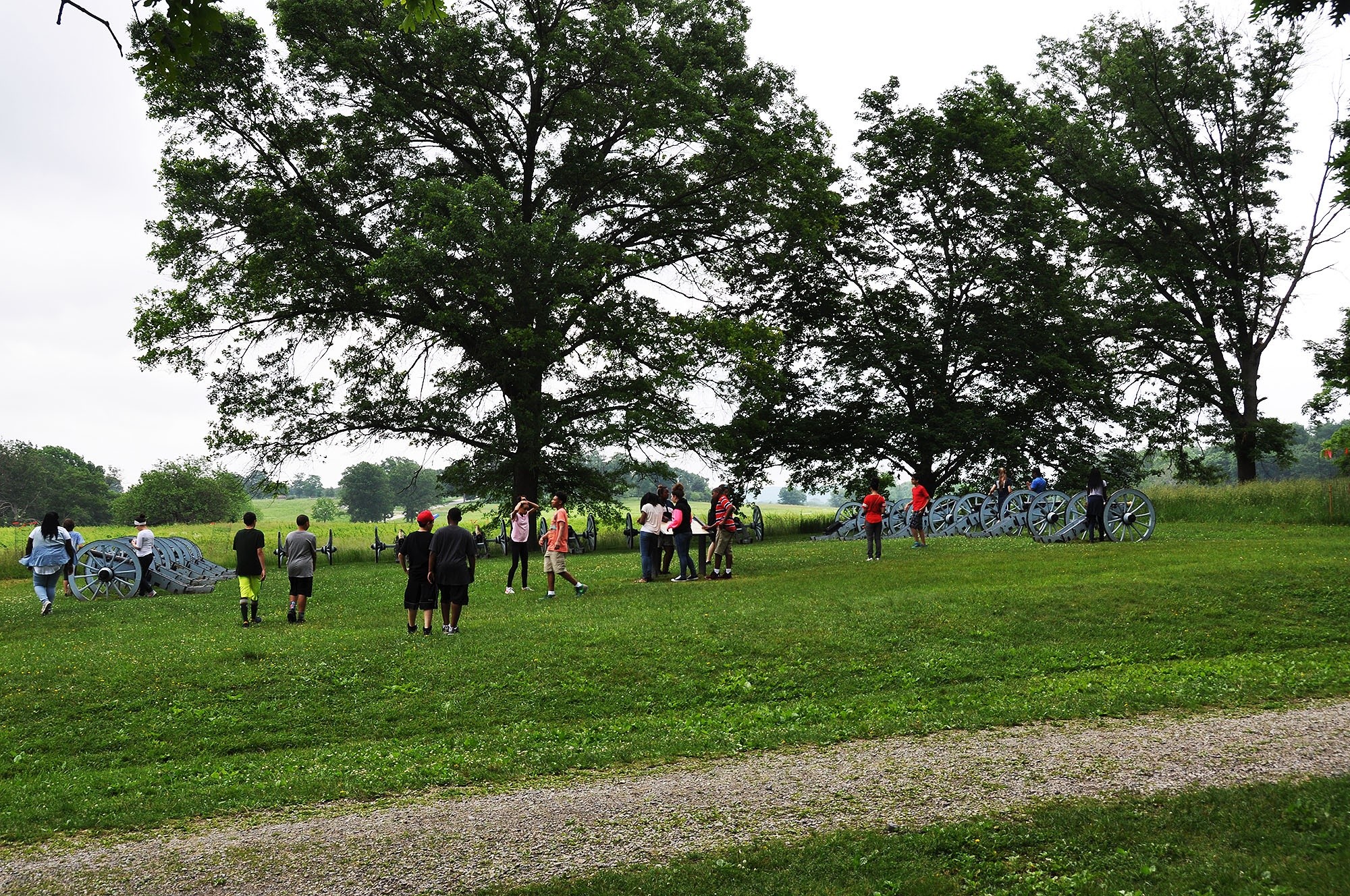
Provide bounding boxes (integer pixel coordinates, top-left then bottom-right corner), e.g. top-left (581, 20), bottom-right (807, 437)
top-left (504, 496), bottom-right (539, 595)
top-left (19, 512), bottom-right (78, 616)
top-left (702, 487), bottom-right (736, 579)
top-left (538, 491), bottom-right (589, 600)
top-left (905, 474), bottom-right (930, 549)
top-left (131, 514), bottom-right (157, 597)
top-left (1026, 468), bottom-right (1047, 501)
top-left (667, 483), bottom-right (699, 582)
top-left (861, 482), bottom-right (886, 562)
top-left (62, 518), bottom-right (85, 597)
top-left (706, 484), bottom-right (733, 565)
top-left (427, 508), bottom-right (476, 636)
top-left (233, 512), bottom-right (266, 628)
top-left (398, 510), bottom-right (439, 636)
top-left (635, 492), bottom-right (664, 582)
top-left (284, 514), bottom-right (317, 622)
top-left (657, 484), bottom-right (675, 575)
top-left (988, 467), bottom-right (1013, 521)
top-left (1086, 468), bottom-right (1107, 543)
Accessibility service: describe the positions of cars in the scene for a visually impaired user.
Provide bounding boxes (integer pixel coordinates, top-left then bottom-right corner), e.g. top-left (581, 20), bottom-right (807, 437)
top-left (11, 516), bottom-right (43, 527)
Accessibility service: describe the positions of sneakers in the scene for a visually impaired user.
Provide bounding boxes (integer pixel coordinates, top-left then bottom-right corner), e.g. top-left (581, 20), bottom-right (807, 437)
top-left (296, 618), bottom-right (306, 623)
top-left (705, 560), bottom-right (711, 564)
top-left (574, 584), bottom-right (588, 596)
top-left (242, 621), bottom-right (250, 629)
top-left (423, 626), bottom-right (432, 635)
top-left (686, 575), bottom-right (699, 582)
top-left (634, 577), bottom-right (647, 583)
top-left (720, 572), bottom-right (732, 579)
top-left (538, 593), bottom-right (556, 601)
top-left (504, 586), bottom-right (515, 594)
top-left (251, 616), bottom-right (261, 624)
top-left (875, 557), bottom-right (880, 561)
top-left (287, 609), bottom-right (297, 623)
top-left (911, 542), bottom-right (920, 549)
top-left (407, 623), bottom-right (418, 635)
top-left (441, 623), bottom-right (451, 633)
top-left (522, 587), bottom-right (535, 592)
top-left (449, 626), bottom-right (459, 635)
top-left (866, 558), bottom-right (873, 562)
top-left (704, 571), bottom-right (721, 580)
top-left (671, 575), bottom-right (686, 582)
top-left (41, 600), bottom-right (51, 616)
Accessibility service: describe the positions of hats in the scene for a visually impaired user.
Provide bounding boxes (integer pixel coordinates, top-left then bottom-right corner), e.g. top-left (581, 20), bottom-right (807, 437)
top-left (417, 510), bottom-right (439, 526)
